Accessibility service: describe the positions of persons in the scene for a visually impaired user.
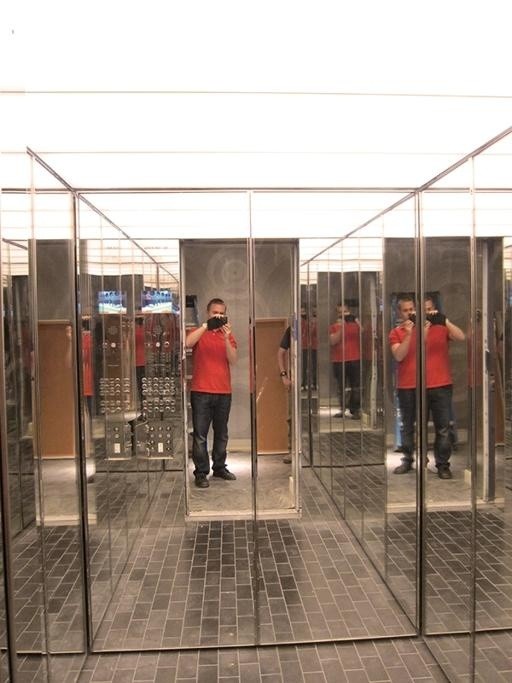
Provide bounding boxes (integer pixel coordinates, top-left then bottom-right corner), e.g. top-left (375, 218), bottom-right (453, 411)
top-left (328, 302), bottom-right (366, 417)
top-left (187, 297), bottom-right (239, 488)
top-left (309, 303), bottom-right (318, 389)
top-left (300, 304), bottom-right (309, 392)
top-left (389, 295), bottom-right (418, 454)
top-left (277, 324), bottom-right (291, 464)
top-left (133, 307), bottom-right (144, 416)
top-left (78, 315), bottom-right (96, 486)
top-left (385, 291), bottom-right (468, 480)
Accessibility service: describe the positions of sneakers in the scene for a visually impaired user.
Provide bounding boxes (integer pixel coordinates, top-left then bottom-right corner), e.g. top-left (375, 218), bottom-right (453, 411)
top-left (193, 471), bottom-right (209, 488)
top-left (213, 469), bottom-right (239, 481)
top-left (335, 409), bottom-right (346, 418)
top-left (436, 465), bottom-right (454, 479)
top-left (393, 459), bottom-right (414, 474)
top-left (283, 454), bottom-right (293, 464)
top-left (352, 412), bottom-right (361, 421)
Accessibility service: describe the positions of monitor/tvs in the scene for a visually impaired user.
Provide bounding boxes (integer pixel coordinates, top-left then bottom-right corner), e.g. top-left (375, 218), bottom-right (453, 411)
top-left (141, 290), bottom-right (171, 312)
top-left (96, 290), bottom-right (128, 315)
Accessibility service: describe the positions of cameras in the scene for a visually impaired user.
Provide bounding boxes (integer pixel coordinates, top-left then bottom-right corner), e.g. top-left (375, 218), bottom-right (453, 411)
top-left (425, 312), bottom-right (446, 326)
top-left (409, 313), bottom-right (417, 325)
top-left (345, 313), bottom-right (356, 322)
top-left (207, 317), bottom-right (227, 330)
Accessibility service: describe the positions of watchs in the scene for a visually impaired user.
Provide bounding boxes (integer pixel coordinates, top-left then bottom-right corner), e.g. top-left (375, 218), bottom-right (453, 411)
top-left (279, 369), bottom-right (287, 377)
